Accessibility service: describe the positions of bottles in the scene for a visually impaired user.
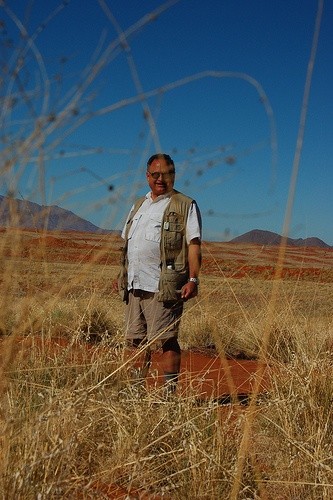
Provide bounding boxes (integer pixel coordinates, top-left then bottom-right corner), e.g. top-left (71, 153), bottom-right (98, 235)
top-left (167, 266), bottom-right (172, 271)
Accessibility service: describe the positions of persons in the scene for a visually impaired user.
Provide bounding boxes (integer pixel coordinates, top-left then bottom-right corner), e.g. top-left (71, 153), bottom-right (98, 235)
top-left (112, 153), bottom-right (203, 390)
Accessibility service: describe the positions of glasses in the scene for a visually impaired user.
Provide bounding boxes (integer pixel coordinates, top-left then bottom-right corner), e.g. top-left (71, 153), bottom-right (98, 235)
top-left (149, 172), bottom-right (168, 179)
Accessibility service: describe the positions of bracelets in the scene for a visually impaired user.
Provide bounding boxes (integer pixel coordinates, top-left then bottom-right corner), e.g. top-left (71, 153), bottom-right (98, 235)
top-left (187, 277), bottom-right (199, 285)
